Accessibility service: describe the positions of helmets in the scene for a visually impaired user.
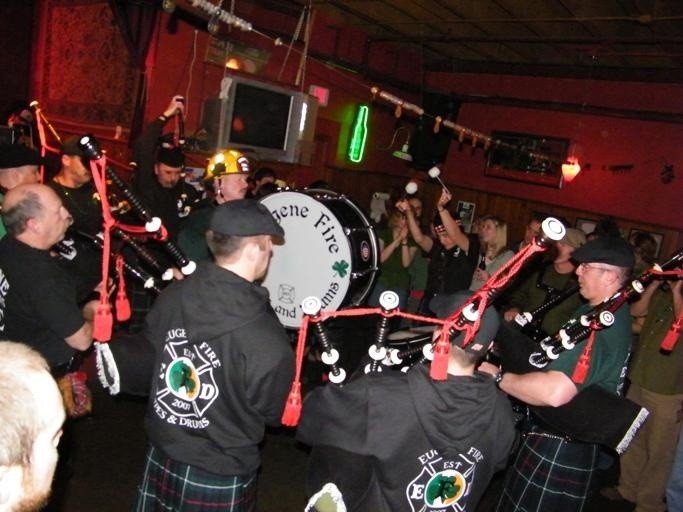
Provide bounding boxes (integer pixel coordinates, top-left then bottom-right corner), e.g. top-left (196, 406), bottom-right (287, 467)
top-left (204, 148), bottom-right (251, 180)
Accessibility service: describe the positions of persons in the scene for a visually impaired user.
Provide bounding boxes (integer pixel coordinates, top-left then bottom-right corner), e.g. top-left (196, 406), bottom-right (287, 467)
top-left (0, 92), bottom-right (683, 512)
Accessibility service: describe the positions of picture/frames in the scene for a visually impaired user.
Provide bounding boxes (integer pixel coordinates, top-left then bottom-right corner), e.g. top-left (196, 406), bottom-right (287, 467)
top-left (627, 227), bottom-right (664, 260)
top-left (456, 200), bottom-right (476, 234)
top-left (573, 217), bottom-right (598, 234)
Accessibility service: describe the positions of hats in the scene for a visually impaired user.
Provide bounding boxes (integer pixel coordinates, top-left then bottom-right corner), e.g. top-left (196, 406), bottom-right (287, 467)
top-left (0, 144), bottom-right (45, 168)
top-left (159, 148), bottom-right (183, 167)
top-left (210, 200), bottom-right (284, 237)
top-left (561, 227), bottom-right (586, 248)
top-left (570, 235), bottom-right (635, 267)
top-left (430, 290), bottom-right (500, 350)
top-left (61, 135), bottom-right (84, 154)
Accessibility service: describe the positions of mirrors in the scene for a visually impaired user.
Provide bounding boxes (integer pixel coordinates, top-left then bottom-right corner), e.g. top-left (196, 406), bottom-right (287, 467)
top-left (483, 130), bottom-right (570, 189)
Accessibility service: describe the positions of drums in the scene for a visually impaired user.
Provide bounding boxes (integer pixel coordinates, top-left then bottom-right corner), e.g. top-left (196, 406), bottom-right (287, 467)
top-left (257, 190), bottom-right (378, 330)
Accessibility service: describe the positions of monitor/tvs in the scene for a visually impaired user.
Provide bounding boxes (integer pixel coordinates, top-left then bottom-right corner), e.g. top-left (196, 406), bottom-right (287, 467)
top-left (200, 74), bottom-right (302, 163)
